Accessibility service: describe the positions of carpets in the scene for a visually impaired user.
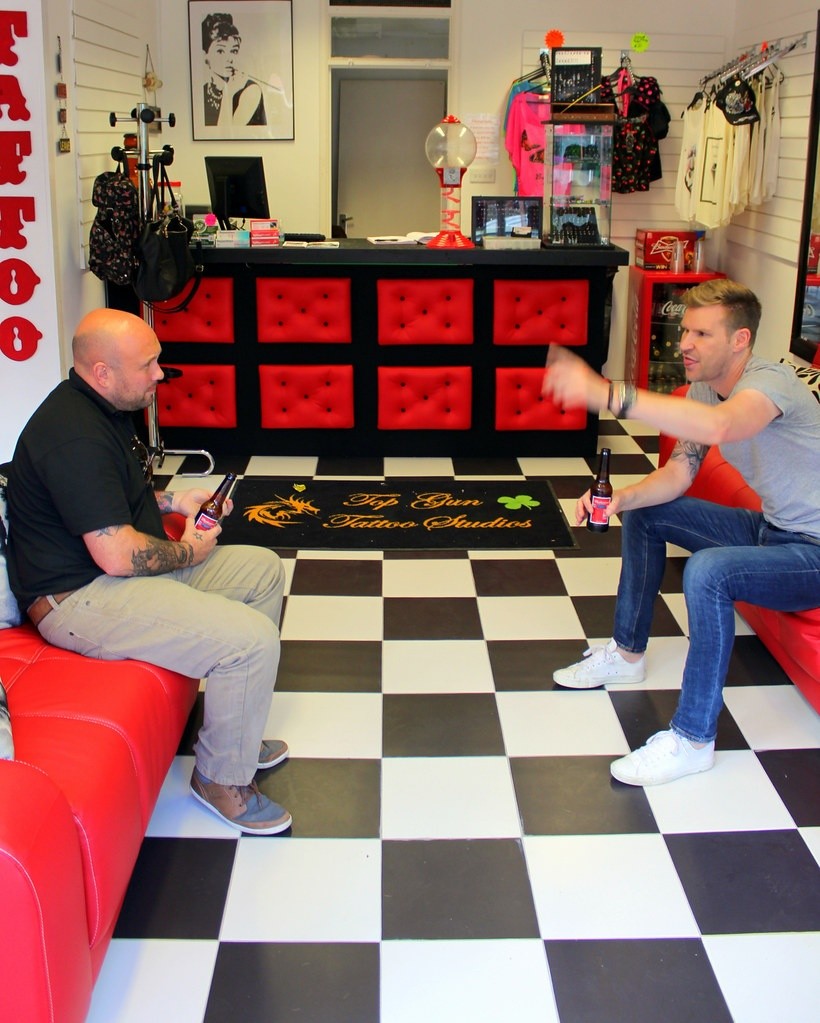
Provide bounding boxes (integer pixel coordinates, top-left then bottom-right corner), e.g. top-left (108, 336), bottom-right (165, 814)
top-left (211, 480), bottom-right (579, 547)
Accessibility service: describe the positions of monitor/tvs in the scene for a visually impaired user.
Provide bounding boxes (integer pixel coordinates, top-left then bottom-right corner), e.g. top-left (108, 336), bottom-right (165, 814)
top-left (205, 155), bottom-right (270, 231)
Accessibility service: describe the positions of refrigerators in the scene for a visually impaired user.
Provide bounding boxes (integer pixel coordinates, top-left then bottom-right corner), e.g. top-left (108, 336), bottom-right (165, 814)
top-left (624, 264), bottom-right (728, 398)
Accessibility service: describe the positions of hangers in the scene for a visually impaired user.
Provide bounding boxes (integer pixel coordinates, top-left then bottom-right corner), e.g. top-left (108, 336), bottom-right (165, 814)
top-left (518, 52), bottom-right (553, 95)
top-left (681, 46), bottom-right (784, 120)
top-left (601, 57), bottom-right (662, 103)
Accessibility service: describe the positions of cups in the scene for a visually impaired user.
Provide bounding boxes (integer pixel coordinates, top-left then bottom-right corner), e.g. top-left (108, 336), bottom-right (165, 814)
top-left (670, 241), bottom-right (683, 273)
top-left (692, 241), bottom-right (705, 273)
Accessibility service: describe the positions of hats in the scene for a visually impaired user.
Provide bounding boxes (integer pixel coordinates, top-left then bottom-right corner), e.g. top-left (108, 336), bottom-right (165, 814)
top-left (716, 78), bottom-right (760, 125)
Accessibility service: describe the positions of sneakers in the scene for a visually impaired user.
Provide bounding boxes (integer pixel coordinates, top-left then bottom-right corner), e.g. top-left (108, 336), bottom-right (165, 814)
top-left (553, 637), bottom-right (645, 689)
top-left (257, 740), bottom-right (289, 769)
top-left (189, 766), bottom-right (293, 835)
top-left (610, 728), bottom-right (714, 786)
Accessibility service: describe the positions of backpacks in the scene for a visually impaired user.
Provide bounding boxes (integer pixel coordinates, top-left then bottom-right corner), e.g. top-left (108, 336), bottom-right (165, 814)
top-left (85, 152), bottom-right (139, 284)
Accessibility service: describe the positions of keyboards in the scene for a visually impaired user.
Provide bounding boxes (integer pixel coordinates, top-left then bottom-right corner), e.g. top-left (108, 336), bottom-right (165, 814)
top-left (285, 233), bottom-right (326, 242)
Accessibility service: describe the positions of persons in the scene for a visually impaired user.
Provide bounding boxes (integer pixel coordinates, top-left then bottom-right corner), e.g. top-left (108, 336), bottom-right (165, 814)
top-left (543, 279), bottom-right (820, 786)
top-left (6, 308), bottom-right (293, 834)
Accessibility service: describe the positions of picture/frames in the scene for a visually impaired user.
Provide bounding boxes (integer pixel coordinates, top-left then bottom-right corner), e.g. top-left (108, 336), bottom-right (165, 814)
top-left (188, 0), bottom-right (294, 140)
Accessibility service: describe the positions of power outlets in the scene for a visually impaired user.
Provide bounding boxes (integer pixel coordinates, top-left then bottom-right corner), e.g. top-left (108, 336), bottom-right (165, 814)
top-left (470, 166), bottom-right (497, 183)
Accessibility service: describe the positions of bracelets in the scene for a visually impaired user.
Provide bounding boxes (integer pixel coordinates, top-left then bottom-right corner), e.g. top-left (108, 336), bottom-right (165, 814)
top-left (604, 380), bottom-right (615, 410)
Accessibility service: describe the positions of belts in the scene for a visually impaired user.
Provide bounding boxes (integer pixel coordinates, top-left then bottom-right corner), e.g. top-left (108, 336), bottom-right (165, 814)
top-left (28, 592), bottom-right (75, 626)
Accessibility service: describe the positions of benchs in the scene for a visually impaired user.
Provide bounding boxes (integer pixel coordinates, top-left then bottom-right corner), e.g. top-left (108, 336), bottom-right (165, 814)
top-left (0, 512), bottom-right (217, 1023)
top-left (656, 381), bottom-right (820, 710)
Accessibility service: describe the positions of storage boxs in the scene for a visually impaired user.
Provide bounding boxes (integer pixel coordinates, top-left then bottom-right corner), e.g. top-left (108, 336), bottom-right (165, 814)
top-left (636, 229), bottom-right (705, 269)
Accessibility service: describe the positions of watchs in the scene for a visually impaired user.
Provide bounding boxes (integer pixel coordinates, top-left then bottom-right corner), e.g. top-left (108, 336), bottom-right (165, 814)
top-left (616, 380), bottom-right (638, 421)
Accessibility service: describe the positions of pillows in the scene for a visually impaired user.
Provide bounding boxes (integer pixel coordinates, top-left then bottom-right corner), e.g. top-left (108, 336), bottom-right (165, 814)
top-left (0, 471), bottom-right (24, 630)
top-left (778, 358), bottom-right (820, 406)
top-left (0, 679), bottom-right (17, 761)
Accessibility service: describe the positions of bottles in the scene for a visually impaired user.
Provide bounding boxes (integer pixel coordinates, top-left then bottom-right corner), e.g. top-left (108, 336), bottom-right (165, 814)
top-left (195, 471), bottom-right (237, 531)
top-left (157, 181), bottom-right (185, 217)
top-left (587, 448), bottom-right (614, 534)
top-left (647, 288), bottom-right (692, 397)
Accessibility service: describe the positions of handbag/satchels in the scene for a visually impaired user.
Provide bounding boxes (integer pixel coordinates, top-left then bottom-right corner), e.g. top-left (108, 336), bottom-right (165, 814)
top-left (133, 154), bottom-right (197, 301)
